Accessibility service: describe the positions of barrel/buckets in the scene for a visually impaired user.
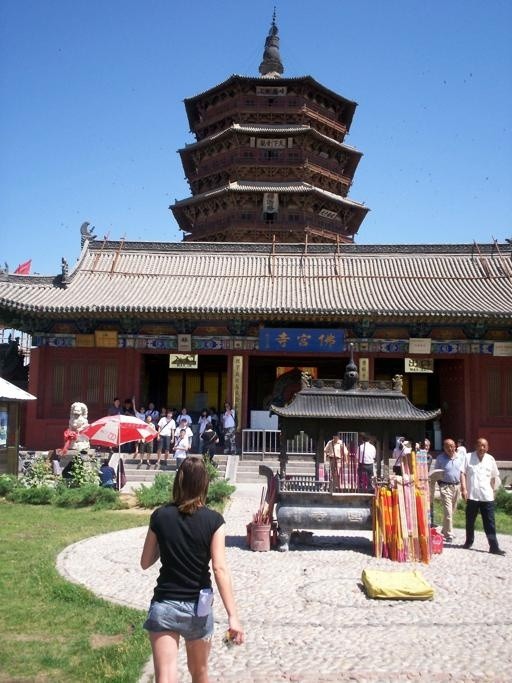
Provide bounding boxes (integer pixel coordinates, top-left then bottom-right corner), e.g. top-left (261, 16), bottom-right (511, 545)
top-left (250, 524), bottom-right (271, 552)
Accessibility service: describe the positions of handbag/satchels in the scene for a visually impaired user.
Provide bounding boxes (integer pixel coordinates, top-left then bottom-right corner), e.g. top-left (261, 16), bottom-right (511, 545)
top-left (393, 465), bottom-right (403, 476)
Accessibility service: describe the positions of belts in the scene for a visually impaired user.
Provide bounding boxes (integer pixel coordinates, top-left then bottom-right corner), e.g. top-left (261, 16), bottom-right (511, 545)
top-left (438, 480), bottom-right (460, 485)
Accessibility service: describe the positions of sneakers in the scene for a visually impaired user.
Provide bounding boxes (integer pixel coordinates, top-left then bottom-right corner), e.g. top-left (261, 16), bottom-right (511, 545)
top-left (440, 529), bottom-right (456, 541)
top-left (488, 546), bottom-right (508, 556)
top-left (462, 535), bottom-right (475, 550)
top-left (132, 452), bottom-right (168, 465)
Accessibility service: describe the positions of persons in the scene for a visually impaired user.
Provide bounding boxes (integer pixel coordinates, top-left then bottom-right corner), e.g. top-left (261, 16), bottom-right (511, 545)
top-left (62, 450), bottom-right (87, 489)
top-left (324, 432), bottom-right (349, 476)
top-left (391, 437), bottom-right (432, 476)
top-left (434, 439), bottom-right (463, 542)
top-left (456, 438), bottom-right (466, 459)
top-left (107, 396), bottom-right (237, 469)
top-left (357, 436), bottom-right (376, 492)
top-left (460, 438), bottom-right (506, 555)
top-left (140, 458), bottom-right (245, 683)
top-left (99, 464), bottom-right (116, 488)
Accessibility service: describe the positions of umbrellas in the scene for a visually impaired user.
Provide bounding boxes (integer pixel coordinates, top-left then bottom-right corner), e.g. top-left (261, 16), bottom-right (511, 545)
top-left (79, 413), bottom-right (160, 491)
